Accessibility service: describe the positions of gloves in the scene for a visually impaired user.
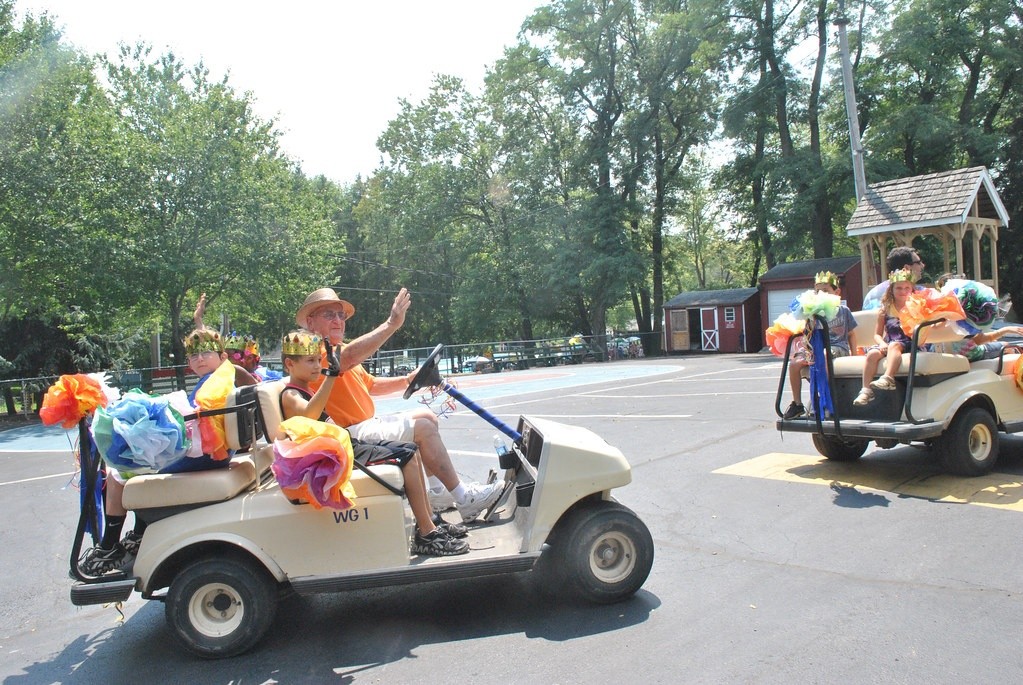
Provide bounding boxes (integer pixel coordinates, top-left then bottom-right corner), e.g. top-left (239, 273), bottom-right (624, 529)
top-left (321, 336), bottom-right (341, 376)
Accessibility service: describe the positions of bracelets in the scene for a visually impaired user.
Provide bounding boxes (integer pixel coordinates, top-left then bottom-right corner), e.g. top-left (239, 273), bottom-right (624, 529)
top-left (405, 375), bottom-right (410, 387)
top-left (997, 330), bottom-right (1001, 337)
top-left (320, 366), bottom-right (339, 377)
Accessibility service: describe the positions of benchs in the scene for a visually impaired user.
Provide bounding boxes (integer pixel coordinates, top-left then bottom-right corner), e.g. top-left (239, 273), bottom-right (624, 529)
top-left (256, 375), bottom-right (405, 505)
top-left (121, 384), bottom-right (274, 522)
top-left (806, 306), bottom-right (971, 378)
top-left (968, 344), bottom-right (1023, 375)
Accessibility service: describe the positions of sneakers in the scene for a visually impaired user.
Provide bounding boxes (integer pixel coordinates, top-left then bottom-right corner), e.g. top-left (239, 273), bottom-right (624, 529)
top-left (455, 480), bottom-right (505, 524)
top-left (870, 375), bottom-right (897, 391)
top-left (853, 388), bottom-right (876, 406)
top-left (427, 481), bottom-right (480, 512)
top-left (784, 401), bottom-right (805, 419)
top-left (119, 529), bottom-right (142, 554)
top-left (415, 513), bottom-right (468, 537)
top-left (68, 541), bottom-right (132, 579)
top-left (411, 530), bottom-right (469, 555)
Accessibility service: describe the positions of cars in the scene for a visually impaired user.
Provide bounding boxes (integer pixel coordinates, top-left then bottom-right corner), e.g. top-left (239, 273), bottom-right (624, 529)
top-left (387, 365), bottom-right (416, 375)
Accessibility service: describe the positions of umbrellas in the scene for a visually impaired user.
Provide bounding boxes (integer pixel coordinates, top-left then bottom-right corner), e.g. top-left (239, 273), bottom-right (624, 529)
top-left (465, 356), bottom-right (489, 364)
top-left (568, 337), bottom-right (586, 346)
top-left (611, 337), bottom-right (628, 348)
top-left (626, 335), bottom-right (641, 344)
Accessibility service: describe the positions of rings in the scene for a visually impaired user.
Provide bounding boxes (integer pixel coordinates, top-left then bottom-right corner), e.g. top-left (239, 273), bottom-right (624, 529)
top-left (1018, 332), bottom-right (1021, 334)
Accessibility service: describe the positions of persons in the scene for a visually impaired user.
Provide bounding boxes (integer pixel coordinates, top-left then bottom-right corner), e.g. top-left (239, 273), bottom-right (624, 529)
top-left (784, 270), bottom-right (858, 421)
top-left (77, 293), bottom-right (262, 581)
top-left (853, 246), bottom-right (1023, 406)
top-left (608, 342), bottom-right (642, 358)
top-left (279, 287), bottom-right (505, 557)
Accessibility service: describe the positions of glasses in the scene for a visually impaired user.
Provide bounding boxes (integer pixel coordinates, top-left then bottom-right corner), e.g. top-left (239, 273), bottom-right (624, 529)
top-left (310, 312), bottom-right (347, 320)
top-left (909, 259), bottom-right (923, 265)
top-left (188, 349), bottom-right (214, 361)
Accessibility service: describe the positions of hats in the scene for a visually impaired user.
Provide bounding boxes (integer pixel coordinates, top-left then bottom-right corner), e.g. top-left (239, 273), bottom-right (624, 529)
top-left (297, 288), bottom-right (355, 329)
